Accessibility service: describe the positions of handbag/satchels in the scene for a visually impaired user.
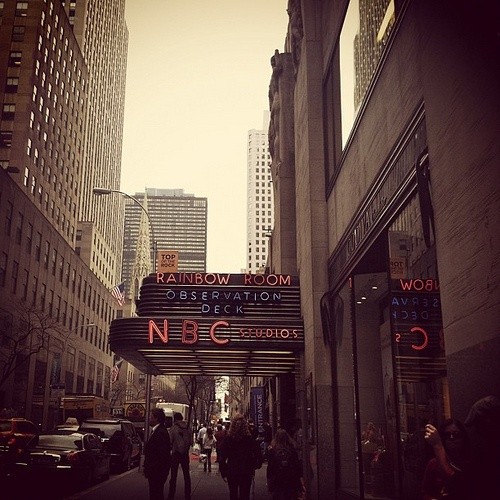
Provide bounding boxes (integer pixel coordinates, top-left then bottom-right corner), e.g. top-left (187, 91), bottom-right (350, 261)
top-left (219, 456), bottom-right (243, 476)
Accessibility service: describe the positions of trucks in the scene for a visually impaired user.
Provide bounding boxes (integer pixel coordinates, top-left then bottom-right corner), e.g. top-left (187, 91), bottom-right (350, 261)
top-left (61, 395), bottom-right (111, 424)
top-left (124, 399), bottom-right (189, 424)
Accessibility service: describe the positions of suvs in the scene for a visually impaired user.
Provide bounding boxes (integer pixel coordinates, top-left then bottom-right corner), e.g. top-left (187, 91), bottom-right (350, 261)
top-left (0, 417), bottom-right (40, 474)
top-left (81, 418), bottom-right (145, 475)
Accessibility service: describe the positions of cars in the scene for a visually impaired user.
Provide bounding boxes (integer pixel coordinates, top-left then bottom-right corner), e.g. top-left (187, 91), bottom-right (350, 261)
top-left (15, 432), bottom-right (111, 496)
top-left (52, 417), bottom-right (81, 436)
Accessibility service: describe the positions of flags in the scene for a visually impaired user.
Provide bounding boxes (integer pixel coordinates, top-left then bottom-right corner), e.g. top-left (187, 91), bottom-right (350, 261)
top-left (112, 361), bottom-right (122, 384)
top-left (112, 283), bottom-right (125, 306)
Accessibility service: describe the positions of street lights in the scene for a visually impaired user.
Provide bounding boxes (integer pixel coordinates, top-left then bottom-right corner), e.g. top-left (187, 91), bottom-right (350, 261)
top-left (91, 187), bottom-right (155, 445)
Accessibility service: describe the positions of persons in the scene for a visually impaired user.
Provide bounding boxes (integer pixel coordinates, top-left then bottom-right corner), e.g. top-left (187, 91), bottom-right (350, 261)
top-left (168, 413), bottom-right (193, 500)
top-left (247, 419), bottom-right (260, 441)
top-left (142, 408), bottom-right (171, 500)
top-left (218, 413), bottom-right (262, 500)
top-left (418, 395), bottom-right (500, 500)
top-left (197, 423), bottom-right (230, 472)
top-left (264, 429), bottom-right (303, 500)
